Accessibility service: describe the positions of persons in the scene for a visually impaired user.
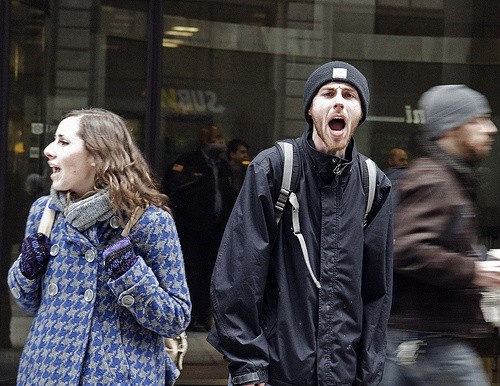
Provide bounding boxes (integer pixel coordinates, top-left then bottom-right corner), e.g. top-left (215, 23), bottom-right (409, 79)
top-left (159, 124), bottom-right (250, 332)
top-left (384, 82), bottom-right (500, 386)
top-left (7, 108), bottom-right (192, 386)
top-left (205, 61), bottom-right (393, 386)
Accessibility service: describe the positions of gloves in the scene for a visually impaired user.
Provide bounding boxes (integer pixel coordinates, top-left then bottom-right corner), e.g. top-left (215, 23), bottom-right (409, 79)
top-left (19, 232), bottom-right (52, 281)
top-left (101, 234), bottom-right (138, 281)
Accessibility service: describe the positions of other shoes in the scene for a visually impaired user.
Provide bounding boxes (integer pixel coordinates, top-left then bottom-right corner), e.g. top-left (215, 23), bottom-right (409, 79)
top-left (185, 320), bottom-right (209, 332)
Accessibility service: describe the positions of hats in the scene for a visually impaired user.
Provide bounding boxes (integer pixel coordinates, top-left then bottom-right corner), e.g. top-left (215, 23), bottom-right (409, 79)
top-left (417, 83), bottom-right (490, 140)
top-left (302, 60), bottom-right (370, 127)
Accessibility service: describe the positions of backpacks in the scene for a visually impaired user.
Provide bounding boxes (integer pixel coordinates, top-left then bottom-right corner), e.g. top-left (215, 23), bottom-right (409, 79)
top-left (35, 194), bottom-right (189, 371)
